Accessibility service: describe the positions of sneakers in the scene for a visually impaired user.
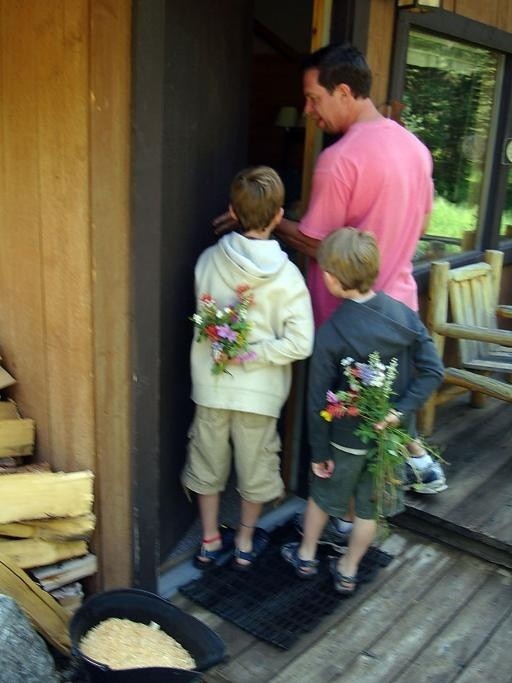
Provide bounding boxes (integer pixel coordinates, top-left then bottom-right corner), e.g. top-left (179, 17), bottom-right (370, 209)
top-left (399, 461), bottom-right (448, 494)
top-left (293, 512), bottom-right (353, 554)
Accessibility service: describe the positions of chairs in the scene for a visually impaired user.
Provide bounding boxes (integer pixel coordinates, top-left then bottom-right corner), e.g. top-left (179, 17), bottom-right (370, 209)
top-left (417, 248), bottom-right (512, 436)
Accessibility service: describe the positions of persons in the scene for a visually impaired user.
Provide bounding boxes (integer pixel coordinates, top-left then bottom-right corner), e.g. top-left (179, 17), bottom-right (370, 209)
top-left (272, 222), bottom-right (444, 601)
top-left (212, 37), bottom-right (450, 557)
top-left (179, 162), bottom-right (315, 569)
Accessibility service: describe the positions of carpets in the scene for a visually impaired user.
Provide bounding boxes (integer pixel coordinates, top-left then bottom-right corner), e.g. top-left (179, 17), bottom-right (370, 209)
top-left (177, 510), bottom-right (392, 651)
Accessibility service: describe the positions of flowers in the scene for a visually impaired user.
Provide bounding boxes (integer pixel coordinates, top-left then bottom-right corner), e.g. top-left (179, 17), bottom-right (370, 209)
top-left (317, 347), bottom-right (451, 537)
top-left (188, 288), bottom-right (255, 373)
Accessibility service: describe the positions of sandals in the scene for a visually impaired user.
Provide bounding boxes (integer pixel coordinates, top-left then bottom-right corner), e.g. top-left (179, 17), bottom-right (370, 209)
top-left (280, 542), bottom-right (319, 579)
top-left (193, 527), bottom-right (269, 570)
top-left (328, 555), bottom-right (357, 595)
